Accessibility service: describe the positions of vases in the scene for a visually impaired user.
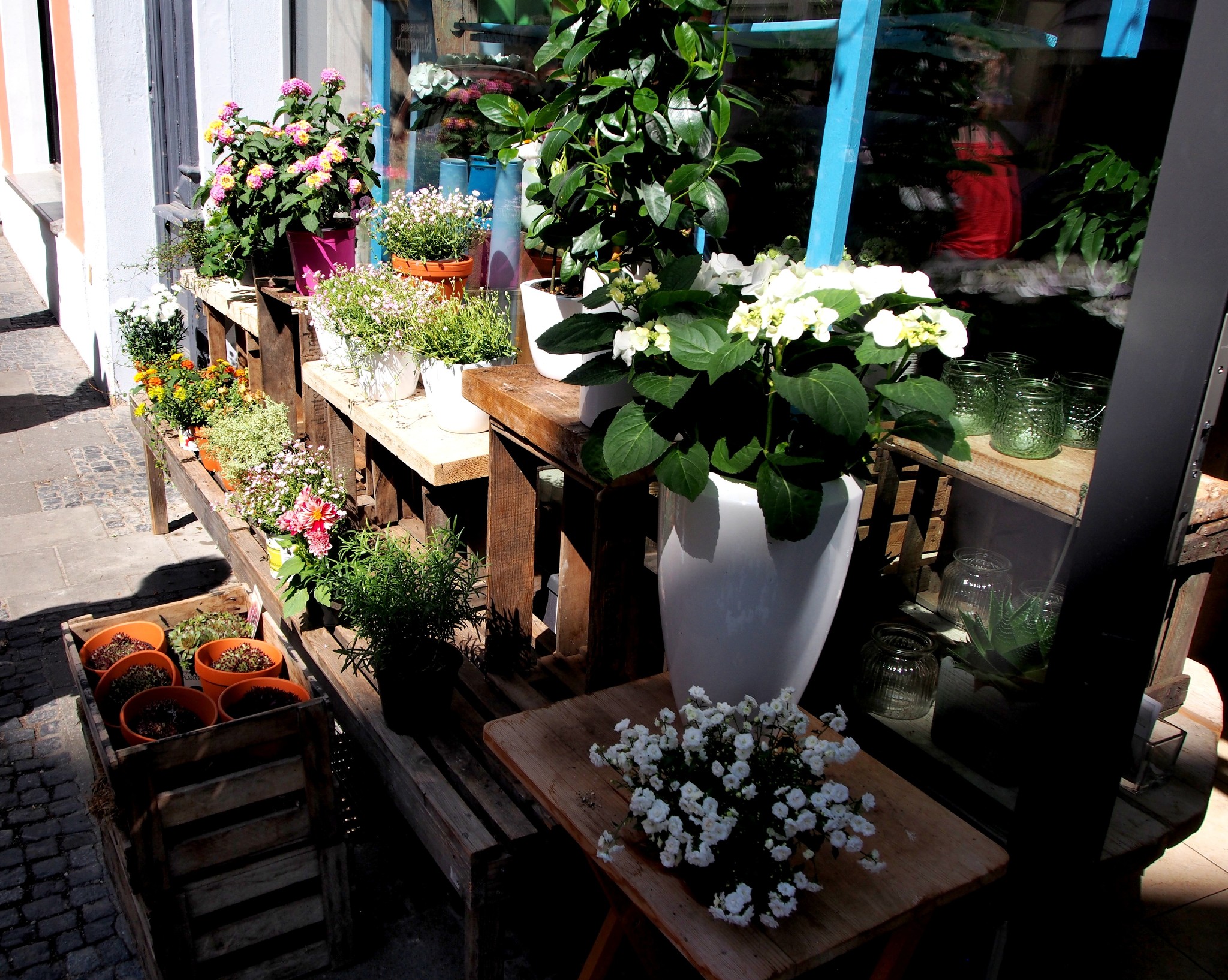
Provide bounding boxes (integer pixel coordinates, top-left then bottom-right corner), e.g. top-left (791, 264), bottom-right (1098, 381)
top-left (650, 460), bottom-right (866, 734)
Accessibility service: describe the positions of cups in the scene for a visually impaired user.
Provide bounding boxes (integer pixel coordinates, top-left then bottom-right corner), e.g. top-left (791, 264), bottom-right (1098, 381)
top-left (940, 352), bottom-right (1113, 459)
top-left (858, 546), bottom-right (1067, 717)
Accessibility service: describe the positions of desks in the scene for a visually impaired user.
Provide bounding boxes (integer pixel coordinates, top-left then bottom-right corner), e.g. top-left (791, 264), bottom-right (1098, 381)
top-left (512, 665), bottom-right (1012, 980)
top-left (133, 394), bottom-right (594, 980)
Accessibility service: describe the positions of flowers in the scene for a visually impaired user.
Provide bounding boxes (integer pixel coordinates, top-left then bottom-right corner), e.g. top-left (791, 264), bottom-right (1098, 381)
top-left (592, 677), bottom-right (881, 939)
top-left (107, 1), bottom-right (974, 652)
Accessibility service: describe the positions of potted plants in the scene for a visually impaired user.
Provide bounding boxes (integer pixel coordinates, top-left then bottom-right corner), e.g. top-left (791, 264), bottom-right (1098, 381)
top-left (118, 686), bottom-right (218, 749)
top-left (82, 621), bottom-right (168, 677)
top-left (221, 676), bottom-right (312, 728)
top-left (193, 642), bottom-right (284, 707)
top-left (309, 517), bottom-right (492, 730)
top-left (96, 649), bottom-right (184, 730)
top-left (169, 610), bottom-right (257, 675)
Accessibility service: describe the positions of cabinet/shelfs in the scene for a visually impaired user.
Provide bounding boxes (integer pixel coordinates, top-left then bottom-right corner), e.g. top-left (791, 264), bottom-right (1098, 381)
top-left (855, 420), bottom-right (1227, 980)
